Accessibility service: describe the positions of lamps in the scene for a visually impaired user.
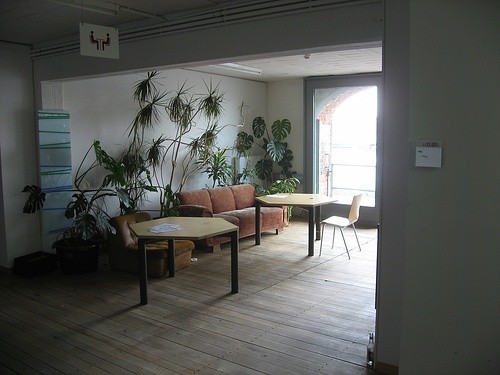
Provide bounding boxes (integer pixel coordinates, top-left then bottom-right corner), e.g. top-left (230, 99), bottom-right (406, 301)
top-left (209, 63), bottom-right (262, 76)
top-left (48, 0), bottom-right (119, 17)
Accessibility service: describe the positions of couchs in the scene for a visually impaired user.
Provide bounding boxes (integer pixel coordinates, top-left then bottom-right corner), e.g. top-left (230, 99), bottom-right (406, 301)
top-left (110, 214), bottom-right (194, 275)
top-left (176, 184), bottom-right (288, 252)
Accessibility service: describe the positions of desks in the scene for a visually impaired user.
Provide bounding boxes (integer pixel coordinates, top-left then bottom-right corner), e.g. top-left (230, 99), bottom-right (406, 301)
top-left (255, 192), bottom-right (339, 256)
top-left (127, 217), bottom-right (240, 304)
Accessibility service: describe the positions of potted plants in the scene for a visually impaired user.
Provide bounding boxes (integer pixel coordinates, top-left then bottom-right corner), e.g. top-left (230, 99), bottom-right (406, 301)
top-left (51, 191), bottom-right (109, 275)
top-left (237, 114), bottom-right (298, 227)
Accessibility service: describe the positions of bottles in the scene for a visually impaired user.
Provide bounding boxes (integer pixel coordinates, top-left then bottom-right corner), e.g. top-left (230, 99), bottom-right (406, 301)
top-left (367, 332), bottom-right (375, 369)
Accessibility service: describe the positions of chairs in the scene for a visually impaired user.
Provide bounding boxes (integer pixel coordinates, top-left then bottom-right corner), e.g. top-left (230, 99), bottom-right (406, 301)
top-left (319, 193), bottom-right (365, 259)
